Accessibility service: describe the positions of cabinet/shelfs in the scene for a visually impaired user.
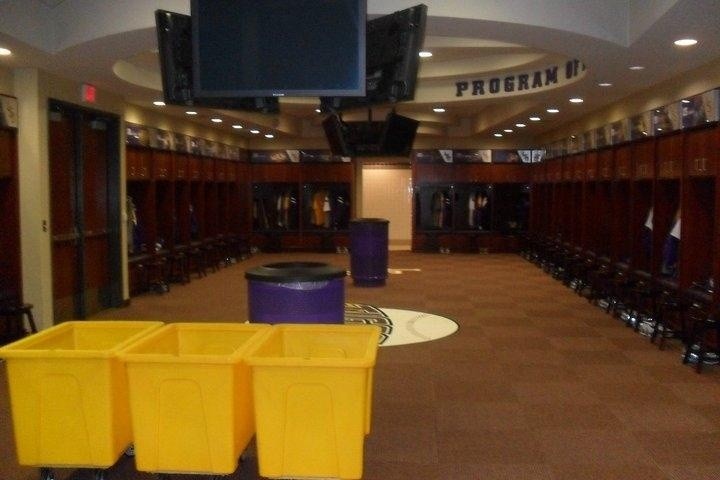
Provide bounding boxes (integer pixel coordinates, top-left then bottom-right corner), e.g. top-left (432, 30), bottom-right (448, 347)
top-left (127, 142), bottom-right (248, 293)
top-left (241, 159), bottom-right (357, 253)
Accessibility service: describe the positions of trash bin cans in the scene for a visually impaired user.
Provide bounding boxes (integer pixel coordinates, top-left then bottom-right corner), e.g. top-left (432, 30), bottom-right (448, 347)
top-left (348, 217), bottom-right (390, 285)
top-left (246, 260), bottom-right (344, 325)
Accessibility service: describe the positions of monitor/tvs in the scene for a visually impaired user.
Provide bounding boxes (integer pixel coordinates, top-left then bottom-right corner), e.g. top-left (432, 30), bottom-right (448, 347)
top-left (322, 113), bottom-right (420, 158)
top-left (321, 4), bottom-right (428, 101)
top-left (155, 9), bottom-right (280, 114)
top-left (190, 0), bottom-right (367, 98)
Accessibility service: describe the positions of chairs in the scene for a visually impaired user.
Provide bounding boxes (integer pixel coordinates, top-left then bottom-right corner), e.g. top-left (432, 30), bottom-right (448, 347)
top-left (523, 228), bottom-right (720, 373)
top-left (133, 231), bottom-right (253, 294)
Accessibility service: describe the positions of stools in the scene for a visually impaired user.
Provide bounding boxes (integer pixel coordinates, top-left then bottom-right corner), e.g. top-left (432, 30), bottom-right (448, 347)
top-left (1, 301), bottom-right (38, 348)
